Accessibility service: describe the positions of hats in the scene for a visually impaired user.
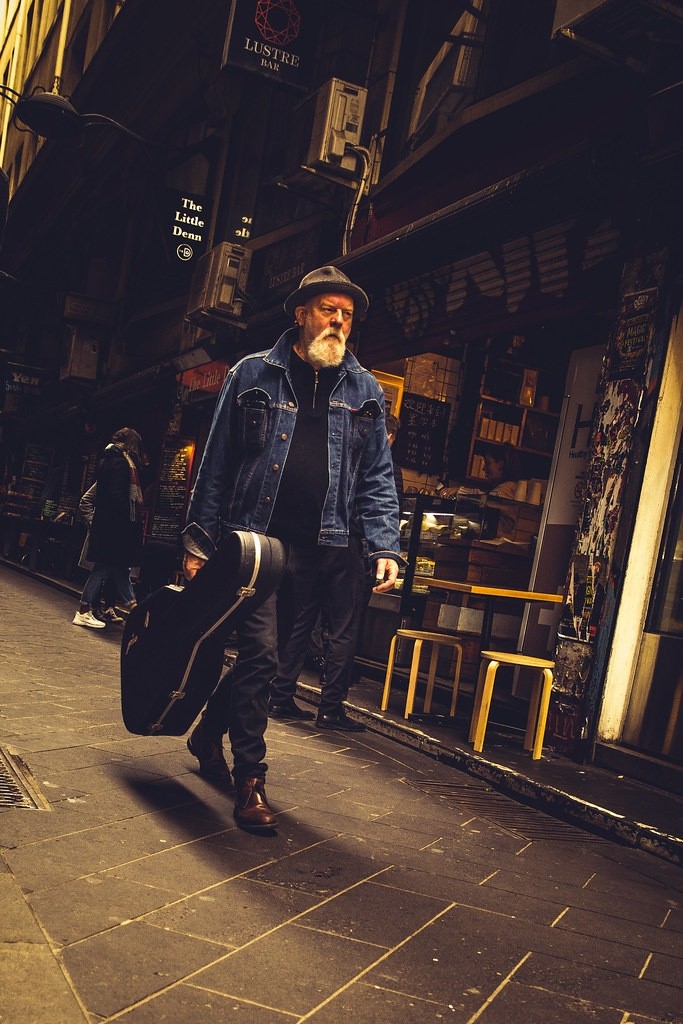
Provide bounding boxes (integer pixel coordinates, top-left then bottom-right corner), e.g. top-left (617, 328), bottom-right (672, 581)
top-left (284, 266), bottom-right (369, 322)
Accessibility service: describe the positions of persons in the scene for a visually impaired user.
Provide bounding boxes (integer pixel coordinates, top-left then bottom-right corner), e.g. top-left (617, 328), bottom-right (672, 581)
top-left (267, 407), bottom-right (402, 731)
top-left (181, 265), bottom-right (408, 832)
top-left (73, 427), bottom-right (151, 629)
top-left (440, 444), bottom-right (518, 498)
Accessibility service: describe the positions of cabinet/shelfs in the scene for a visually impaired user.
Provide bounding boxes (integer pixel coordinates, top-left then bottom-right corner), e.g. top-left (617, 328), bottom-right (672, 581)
top-left (353, 494), bottom-right (501, 669)
top-left (451, 349), bottom-right (566, 487)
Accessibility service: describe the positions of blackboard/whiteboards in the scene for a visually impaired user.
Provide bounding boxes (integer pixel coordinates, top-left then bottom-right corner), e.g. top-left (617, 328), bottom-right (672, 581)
top-left (146, 435), bottom-right (197, 546)
top-left (19, 445), bottom-right (100, 526)
top-left (394, 392), bottom-right (452, 475)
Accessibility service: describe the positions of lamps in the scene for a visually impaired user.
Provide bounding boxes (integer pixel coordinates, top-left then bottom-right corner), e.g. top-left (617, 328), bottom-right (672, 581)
top-left (16, 92), bottom-right (163, 149)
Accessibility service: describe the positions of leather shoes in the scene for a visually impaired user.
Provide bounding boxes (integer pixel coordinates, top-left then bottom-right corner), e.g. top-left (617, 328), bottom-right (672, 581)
top-left (268, 698), bottom-right (314, 720)
top-left (318, 710), bottom-right (368, 732)
top-left (187, 734), bottom-right (232, 789)
top-left (234, 777), bottom-right (279, 831)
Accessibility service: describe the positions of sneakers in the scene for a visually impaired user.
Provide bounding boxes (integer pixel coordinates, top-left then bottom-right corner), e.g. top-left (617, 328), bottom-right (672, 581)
top-left (100, 608), bottom-right (123, 622)
top-left (72, 611), bottom-right (105, 628)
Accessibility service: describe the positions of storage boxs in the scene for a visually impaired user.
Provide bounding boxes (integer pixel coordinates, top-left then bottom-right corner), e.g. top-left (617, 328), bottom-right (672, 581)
top-left (479, 418), bottom-right (520, 445)
top-left (470, 454), bottom-right (486, 479)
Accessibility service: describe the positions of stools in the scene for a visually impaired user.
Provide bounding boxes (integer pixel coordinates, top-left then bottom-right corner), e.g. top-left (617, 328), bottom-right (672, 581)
top-left (467, 651), bottom-right (556, 761)
top-left (380, 629), bottom-right (462, 719)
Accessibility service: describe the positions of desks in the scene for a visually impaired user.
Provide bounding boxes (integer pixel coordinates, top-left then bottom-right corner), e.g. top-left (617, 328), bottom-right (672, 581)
top-left (20, 518), bottom-right (68, 571)
top-left (413, 575), bottom-right (564, 713)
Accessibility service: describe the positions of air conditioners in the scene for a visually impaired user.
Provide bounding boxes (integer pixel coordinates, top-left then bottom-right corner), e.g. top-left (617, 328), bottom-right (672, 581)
top-left (283, 77), bottom-right (368, 194)
top-left (59, 331), bottom-right (100, 381)
top-left (187, 241), bottom-right (253, 326)
